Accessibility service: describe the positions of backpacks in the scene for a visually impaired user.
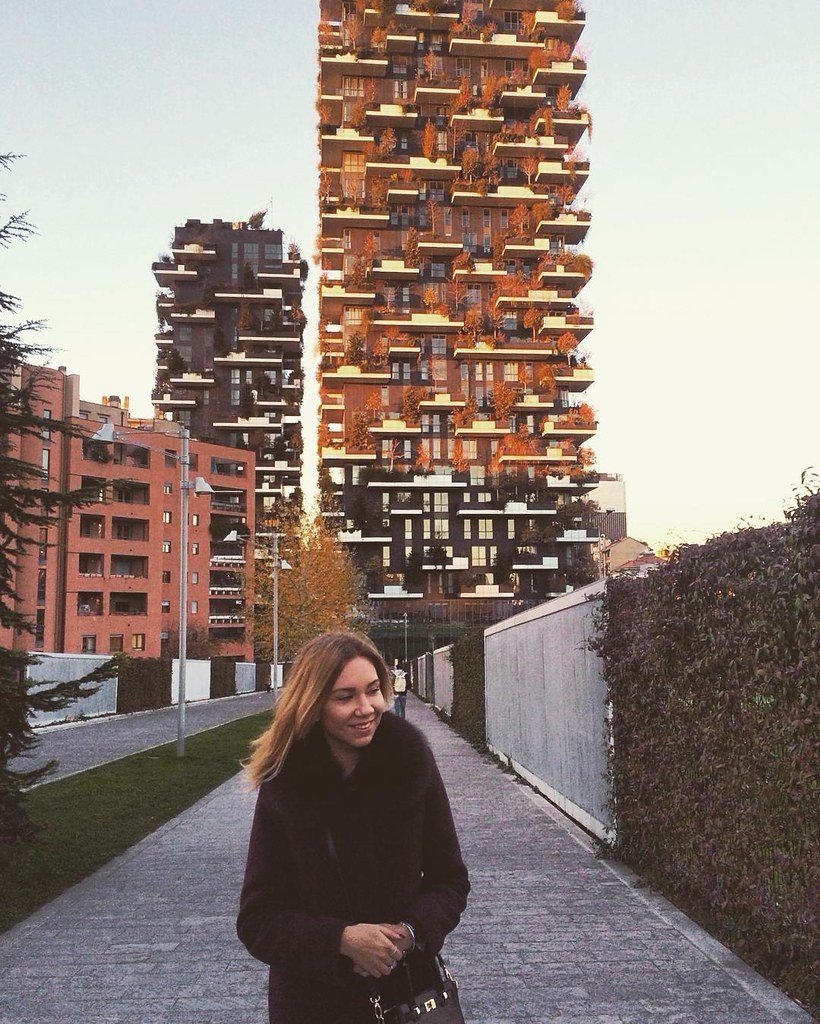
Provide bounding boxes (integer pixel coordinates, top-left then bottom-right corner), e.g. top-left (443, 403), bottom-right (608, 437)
top-left (394, 673), bottom-right (406, 692)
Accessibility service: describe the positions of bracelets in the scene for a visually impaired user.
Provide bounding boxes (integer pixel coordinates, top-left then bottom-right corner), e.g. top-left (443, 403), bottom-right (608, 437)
top-left (401, 920), bottom-right (415, 951)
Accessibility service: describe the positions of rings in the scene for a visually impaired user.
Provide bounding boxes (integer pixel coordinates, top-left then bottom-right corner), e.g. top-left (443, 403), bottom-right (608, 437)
top-left (391, 946), bottom-right (397, 956)
top-left (391, 961), bottom-right (396, 969)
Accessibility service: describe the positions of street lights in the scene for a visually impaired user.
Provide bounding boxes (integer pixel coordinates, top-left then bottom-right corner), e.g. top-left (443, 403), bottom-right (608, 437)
top-left (222, 530), bottom-right (292, 702)
top-left (87, 423), bottom-right (214, 758)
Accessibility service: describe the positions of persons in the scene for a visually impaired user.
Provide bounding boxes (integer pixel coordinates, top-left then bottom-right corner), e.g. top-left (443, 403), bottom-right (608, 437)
top-left (237, 632), bottom-right (470, 1024)
top-left (264, 675), bottom-right (271, 692)
top-left (391, 664), bottom-right (410, 720)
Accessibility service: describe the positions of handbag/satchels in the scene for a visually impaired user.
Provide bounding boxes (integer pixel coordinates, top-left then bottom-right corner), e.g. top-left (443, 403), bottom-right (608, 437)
top-left (369, 947), bottom-right (464, 1024)
